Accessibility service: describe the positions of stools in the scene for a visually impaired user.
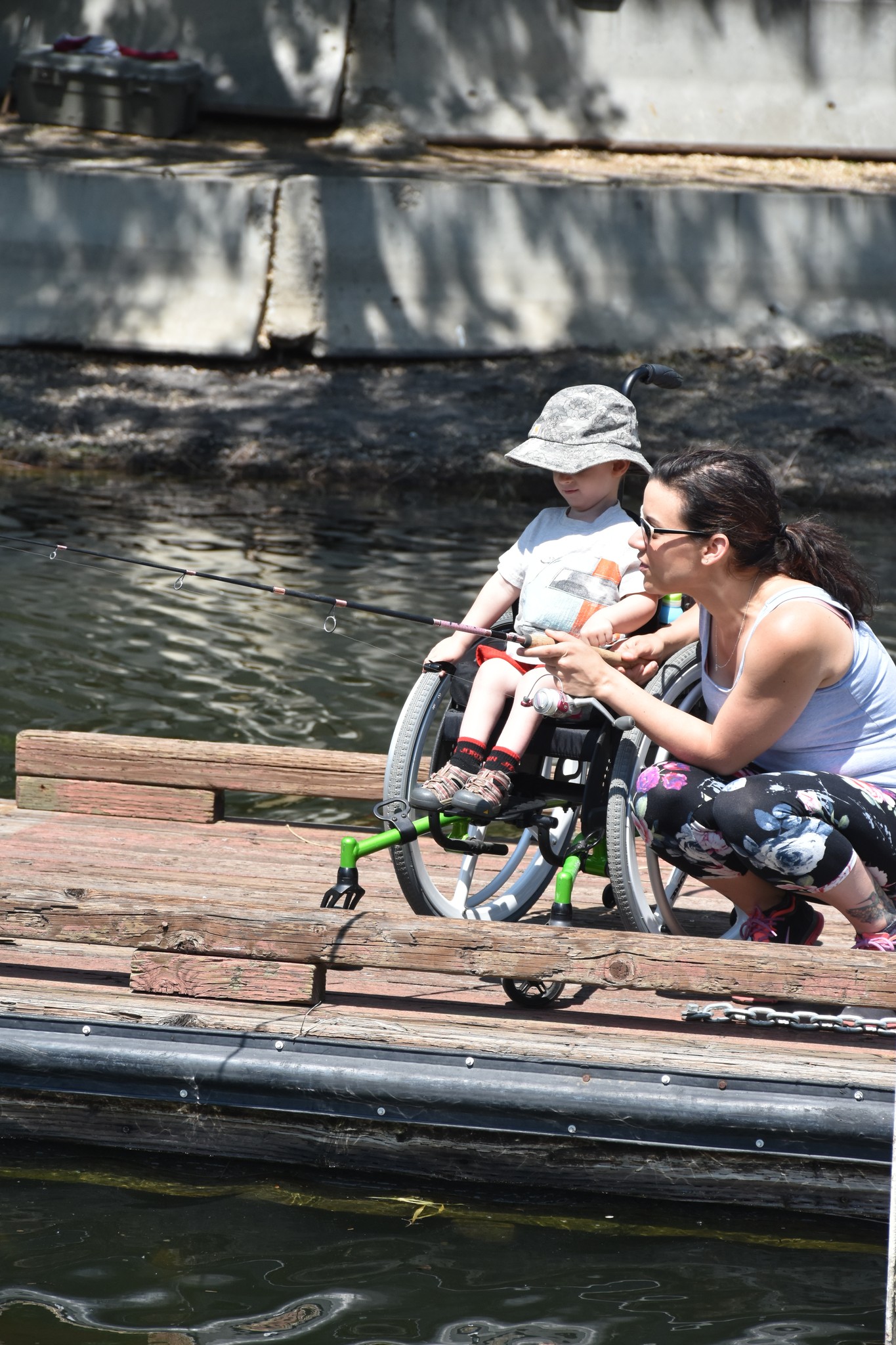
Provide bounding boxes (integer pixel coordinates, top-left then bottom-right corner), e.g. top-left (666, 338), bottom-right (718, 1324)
top-left (7, 39), bottom-right (204, 143)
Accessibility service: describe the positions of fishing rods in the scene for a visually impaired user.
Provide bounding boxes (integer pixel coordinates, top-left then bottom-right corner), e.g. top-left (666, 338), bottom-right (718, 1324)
top-left (1, 532), bottom-right (649, 669)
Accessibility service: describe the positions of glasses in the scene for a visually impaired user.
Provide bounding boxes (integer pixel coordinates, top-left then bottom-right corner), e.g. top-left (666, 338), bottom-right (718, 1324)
top-left (639, 503), bottom-right (752, 553)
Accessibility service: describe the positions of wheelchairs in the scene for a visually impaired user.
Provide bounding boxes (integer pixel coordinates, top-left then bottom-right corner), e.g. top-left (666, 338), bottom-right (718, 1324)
top-left (320, 591), bottom-right (750, 1011)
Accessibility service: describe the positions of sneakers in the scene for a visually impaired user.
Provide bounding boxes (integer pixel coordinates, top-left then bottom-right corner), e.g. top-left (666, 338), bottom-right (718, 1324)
top-left (850, 900), bottom-right (896, 952)
top-left (729, 891), bottom-right (825, 1004)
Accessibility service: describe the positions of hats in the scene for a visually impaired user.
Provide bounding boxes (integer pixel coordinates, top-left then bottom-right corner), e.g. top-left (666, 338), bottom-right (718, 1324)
top-left (503, 385), bottom-right (654, 476)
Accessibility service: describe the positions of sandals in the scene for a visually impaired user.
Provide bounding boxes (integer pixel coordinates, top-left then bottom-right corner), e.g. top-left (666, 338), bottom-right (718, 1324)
top-left (451, 761), bottom-right (514, 819)
top-left (411, 759), bottom-right (478, 813)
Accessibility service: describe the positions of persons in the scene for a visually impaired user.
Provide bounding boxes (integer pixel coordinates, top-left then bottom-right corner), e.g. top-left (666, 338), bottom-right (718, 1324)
top-left (516, 444), bottom-right (896, 951)
top-left (409, 383), bottom-right (659, 819)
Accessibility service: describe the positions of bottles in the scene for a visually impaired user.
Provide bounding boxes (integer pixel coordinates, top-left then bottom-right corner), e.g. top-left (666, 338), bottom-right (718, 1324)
top-left (658, 593), bottom-right (683, 624)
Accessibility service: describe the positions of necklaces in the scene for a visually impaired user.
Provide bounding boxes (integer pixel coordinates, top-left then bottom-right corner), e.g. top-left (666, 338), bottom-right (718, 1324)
top-left (714, 577), bottom-right (759, 673)
